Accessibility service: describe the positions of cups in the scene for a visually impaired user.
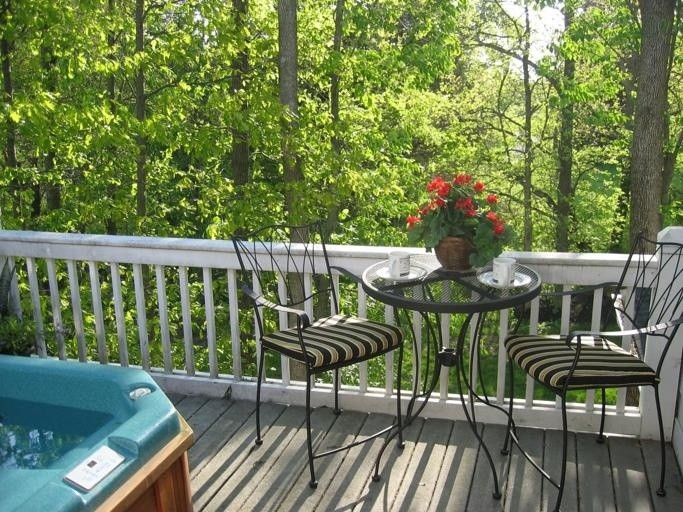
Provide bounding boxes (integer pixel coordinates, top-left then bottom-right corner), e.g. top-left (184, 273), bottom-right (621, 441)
top-left (386, 250), bottom-right (410, 276)
top-left (492, 255), bottom-right (517, 287)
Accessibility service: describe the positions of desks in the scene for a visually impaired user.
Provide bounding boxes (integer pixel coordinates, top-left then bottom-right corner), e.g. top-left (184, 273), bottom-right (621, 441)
top-left (360, 247), bottom-right (543, 502)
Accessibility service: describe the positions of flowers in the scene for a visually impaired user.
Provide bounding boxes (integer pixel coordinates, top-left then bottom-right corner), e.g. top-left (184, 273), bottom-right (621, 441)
top-left (398, 171), bottom-right (509, 270)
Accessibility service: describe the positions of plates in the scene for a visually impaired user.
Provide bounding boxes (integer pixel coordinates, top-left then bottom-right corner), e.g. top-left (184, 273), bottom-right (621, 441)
top-left (477, 270), bottom-right (533, 289)
top-left (377, 264), bottom-right (428, 284)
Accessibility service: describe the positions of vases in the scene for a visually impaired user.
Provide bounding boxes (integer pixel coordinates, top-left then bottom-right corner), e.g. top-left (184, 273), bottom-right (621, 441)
top-left (434, 237), bottom-right (472, 273)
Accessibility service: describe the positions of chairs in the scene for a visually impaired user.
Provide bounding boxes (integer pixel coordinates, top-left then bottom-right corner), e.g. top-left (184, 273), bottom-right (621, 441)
top-left (500, 230), bottom-right (683, 511)
top-left (227, 217), bottom-right (405, 491)
